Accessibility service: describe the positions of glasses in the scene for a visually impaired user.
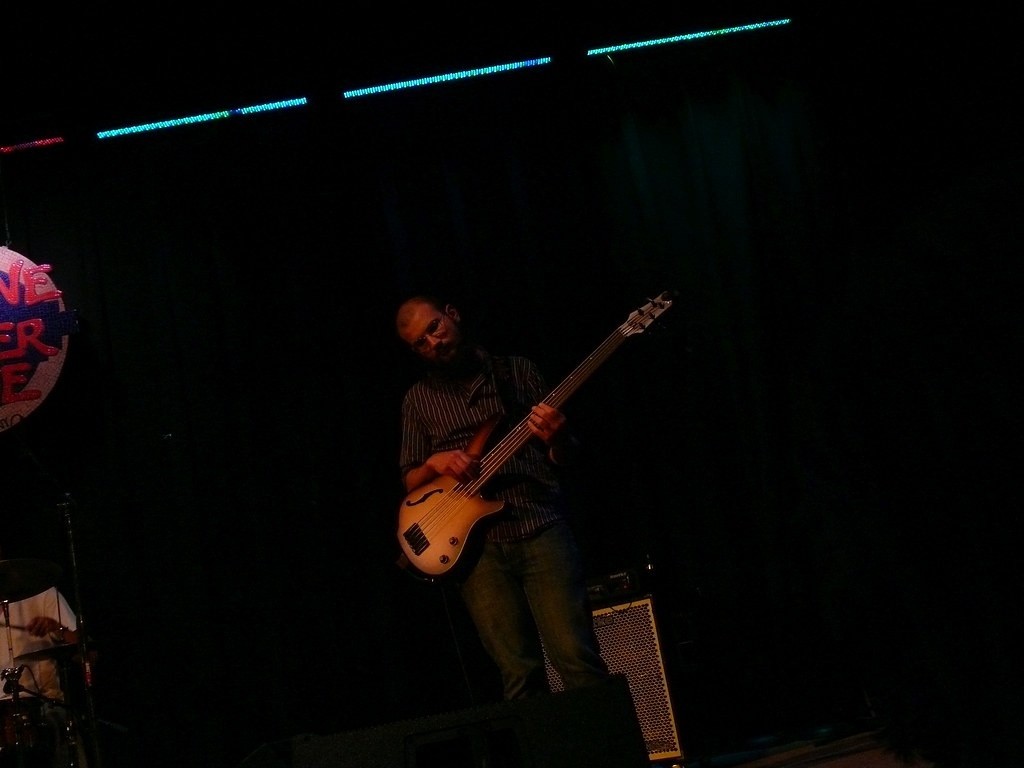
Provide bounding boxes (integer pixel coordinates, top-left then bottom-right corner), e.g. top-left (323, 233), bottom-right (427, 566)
top-left (411, 312), bottom-right (448, 355)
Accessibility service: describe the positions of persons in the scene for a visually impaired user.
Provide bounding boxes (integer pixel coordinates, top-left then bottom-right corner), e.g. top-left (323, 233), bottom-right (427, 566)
top-left (0, 587), bottom-right (77, 701)
top-left (396, 297), bottom-right (609, 702)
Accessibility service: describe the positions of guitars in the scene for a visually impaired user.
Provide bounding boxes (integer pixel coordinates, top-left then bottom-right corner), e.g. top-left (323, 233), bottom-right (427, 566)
top-left (394, 290), bottom-right (672, 578)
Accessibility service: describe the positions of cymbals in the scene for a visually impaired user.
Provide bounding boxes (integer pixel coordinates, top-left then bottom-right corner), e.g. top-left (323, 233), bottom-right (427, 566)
top-left (0, 558), bottom-right (64, 604)
top-left (13, 639), bottom-right (109, 662)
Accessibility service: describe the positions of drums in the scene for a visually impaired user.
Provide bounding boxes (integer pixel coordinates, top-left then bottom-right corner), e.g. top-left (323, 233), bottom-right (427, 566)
top-left (0, 696), bottom-right (44, 750)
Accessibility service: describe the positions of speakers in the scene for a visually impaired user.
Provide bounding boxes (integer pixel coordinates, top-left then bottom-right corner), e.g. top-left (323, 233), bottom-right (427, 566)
top-left (530, 552), bottom-right (691, 767)
